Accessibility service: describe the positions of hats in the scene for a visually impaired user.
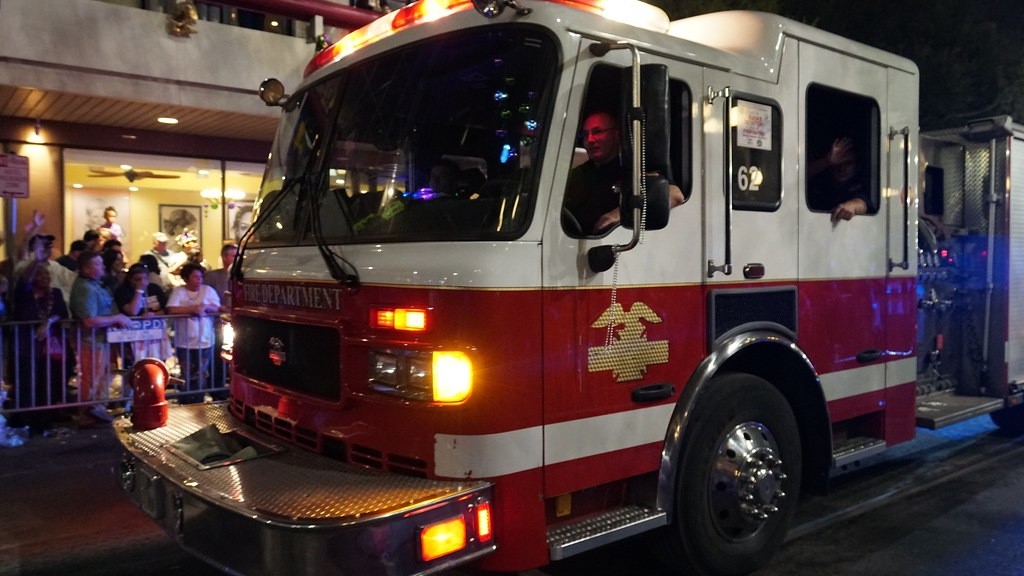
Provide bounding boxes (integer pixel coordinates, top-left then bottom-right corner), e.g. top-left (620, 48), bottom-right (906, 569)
top-left (152, 231), bottom-right (168, 243)
top-left (180, 233), bottom-right (197, 246)
top-left (28, 233), bottom-right (56, 251)
top-left (127, 263), bottom-right (151, 277)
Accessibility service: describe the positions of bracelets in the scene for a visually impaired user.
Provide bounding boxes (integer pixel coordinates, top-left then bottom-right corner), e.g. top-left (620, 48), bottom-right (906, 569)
top-left (48, 317), bottom-right (55, 323)
top-left (135, 289), bottom-right (145, 294)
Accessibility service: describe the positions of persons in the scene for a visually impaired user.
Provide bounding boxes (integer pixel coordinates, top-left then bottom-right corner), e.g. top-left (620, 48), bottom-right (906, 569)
top-left (0, 207), bottom-right (237, 424)
top-left (393, 159), bottom-right (472, 199)
top-left (807, 137), bottom-right (870, 221)
top-left (562, 109), bottom-right (684, 235)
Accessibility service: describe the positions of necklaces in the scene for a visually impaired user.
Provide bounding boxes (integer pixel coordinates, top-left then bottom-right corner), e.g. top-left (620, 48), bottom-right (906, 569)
top-left (185, 285), bottom-right (200, 300)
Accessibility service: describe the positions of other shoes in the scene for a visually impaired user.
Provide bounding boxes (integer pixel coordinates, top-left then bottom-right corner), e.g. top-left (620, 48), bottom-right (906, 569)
top-left (78, 423), bottom-right (104, 429)
top-left (204, 394), bottom-right (213, 404)
top-left (87, 404), bottom-right (113, 423)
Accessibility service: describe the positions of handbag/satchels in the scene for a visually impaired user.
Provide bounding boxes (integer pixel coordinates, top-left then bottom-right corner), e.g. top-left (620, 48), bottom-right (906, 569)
top-left (130, 313), bottom-right (173, 364)
top-left (42, 335), bottom-right (75, 365)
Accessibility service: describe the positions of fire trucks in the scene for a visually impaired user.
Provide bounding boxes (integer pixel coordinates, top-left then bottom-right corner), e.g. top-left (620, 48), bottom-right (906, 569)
top-left (110, 0), bottom-right (1024, 576)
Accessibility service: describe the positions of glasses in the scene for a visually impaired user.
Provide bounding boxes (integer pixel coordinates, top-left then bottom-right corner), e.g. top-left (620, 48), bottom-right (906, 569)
top-left (582, 126), bottom-right (618, 139)
